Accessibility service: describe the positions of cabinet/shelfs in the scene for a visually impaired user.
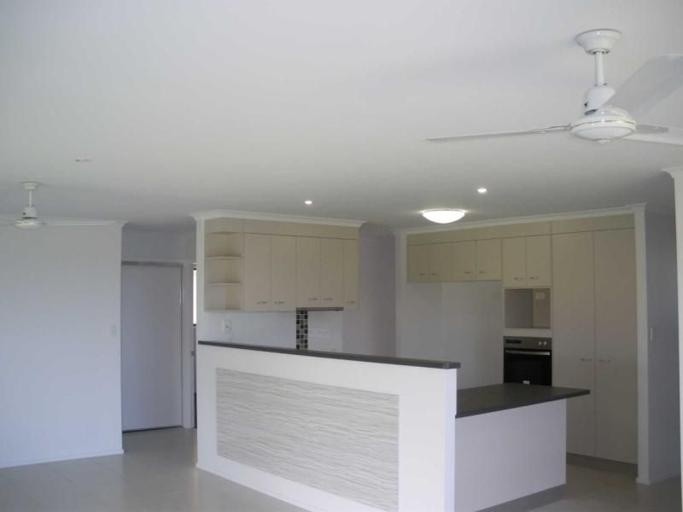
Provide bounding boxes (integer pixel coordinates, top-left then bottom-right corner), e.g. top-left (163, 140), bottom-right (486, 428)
top-left (204, 218), bottom-right (242, 312)
top-left (407, 230), bottom-right (449, 283)
top-left (242, 220), bottom-right (296, 312)
top-left (548, 207), bottom-right (637, 466)
top-left (501, 223), bottom-right (552, 290)
top-left (296, 225), bottom-right (357, 311)
top-left (449, 226), bottom-right (501, 282)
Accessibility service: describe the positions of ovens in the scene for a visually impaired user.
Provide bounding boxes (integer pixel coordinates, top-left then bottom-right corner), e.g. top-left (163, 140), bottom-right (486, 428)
top-left (503, 337), bottom-right (553, 387)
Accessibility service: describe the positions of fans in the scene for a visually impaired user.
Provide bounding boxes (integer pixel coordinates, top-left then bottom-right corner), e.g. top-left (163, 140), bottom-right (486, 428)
top-left (0, 181), bottom-right (121, 234)
top-left (427, 27), bottom-right (683, 155)
